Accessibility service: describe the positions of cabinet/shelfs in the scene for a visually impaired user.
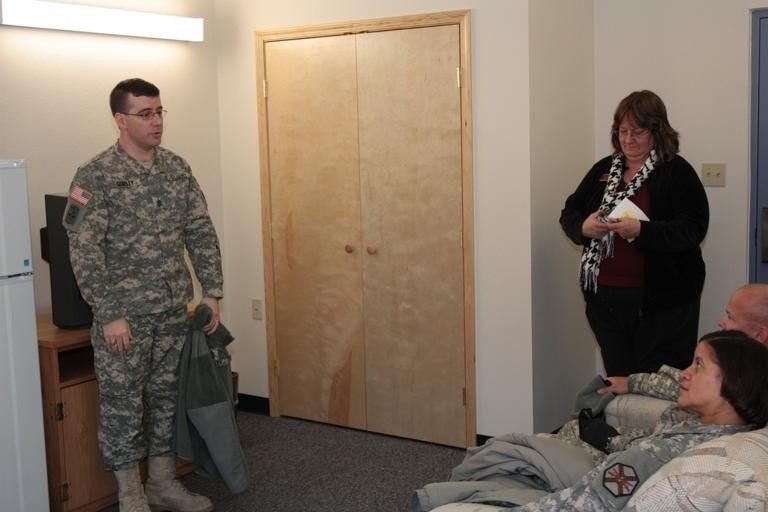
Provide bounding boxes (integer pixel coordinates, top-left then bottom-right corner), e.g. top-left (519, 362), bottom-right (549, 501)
top-left (36, 310), bottom-right (196, 512)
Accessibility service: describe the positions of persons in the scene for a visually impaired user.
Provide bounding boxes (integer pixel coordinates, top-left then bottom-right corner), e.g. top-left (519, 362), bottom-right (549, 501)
top-left (528, 281), bottom-right (768, 470)
top-left (555, 87), bottom-right (712, 399)
top-left (424, 327), bottom-right (767, 512)
top-left (61, 78), bottom-right (228, 512)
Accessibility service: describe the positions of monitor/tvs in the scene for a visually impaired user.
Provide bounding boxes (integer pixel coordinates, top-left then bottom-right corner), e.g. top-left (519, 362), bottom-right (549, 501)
top-left (40, 192), bottom-right (94, 330)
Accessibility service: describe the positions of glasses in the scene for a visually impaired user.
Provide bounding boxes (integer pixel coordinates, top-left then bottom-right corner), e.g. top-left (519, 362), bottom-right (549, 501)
top-left (118, 109), bottom-right (168, 121)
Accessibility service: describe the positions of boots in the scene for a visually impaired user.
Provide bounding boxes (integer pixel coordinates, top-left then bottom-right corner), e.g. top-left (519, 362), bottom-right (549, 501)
top-left (112, 461), bottom-right (153, 512)
top-left (144, 455), bottom-right (215, 512)
